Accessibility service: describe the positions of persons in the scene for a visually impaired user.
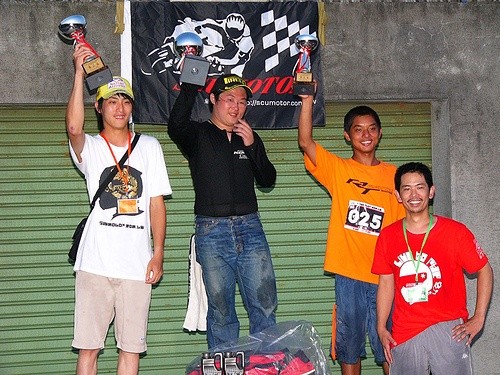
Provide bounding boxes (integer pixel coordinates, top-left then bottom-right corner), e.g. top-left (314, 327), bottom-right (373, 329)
top-left (372, 162), bottom-right (494, 375)
top-left (66, 45), bottom-right (172, 375)
top-left (167, 59), bottom-right (277, 351)
top-left (298, 77), bottom-right (406, 375)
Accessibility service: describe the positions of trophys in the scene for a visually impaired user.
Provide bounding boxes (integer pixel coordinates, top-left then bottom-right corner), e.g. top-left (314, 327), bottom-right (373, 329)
top-left (175, 32), bottom-right (210, 87)
top-left (58, 15), bottom-right (112, 90)
top-left (293, 34), bottom-right (319, 95)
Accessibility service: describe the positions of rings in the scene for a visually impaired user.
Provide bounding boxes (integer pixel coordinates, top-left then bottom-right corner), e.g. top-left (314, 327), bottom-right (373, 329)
top-left (463, 327), bottom-right (466, 331)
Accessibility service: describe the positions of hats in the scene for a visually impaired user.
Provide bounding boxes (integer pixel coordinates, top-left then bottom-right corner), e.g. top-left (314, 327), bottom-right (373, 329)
top-left (210, 73), bottom-right (253, 97)
top-left (97, 76), bottom-right (134, 101)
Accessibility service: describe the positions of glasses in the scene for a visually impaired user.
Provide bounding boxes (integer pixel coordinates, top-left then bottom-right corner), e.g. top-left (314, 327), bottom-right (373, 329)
top-left (219, 97), bottom-right (248, 108)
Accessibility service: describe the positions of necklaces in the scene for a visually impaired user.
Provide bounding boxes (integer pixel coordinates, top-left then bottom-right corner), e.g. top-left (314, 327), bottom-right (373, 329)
top-left (208, 118), bottom-right (232, 133)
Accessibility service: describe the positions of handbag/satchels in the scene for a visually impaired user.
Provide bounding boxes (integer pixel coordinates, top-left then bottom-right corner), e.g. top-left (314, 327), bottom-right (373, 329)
top-left (68, 218), bottom-right (88, 260)
top-left (186, 348), bottom-right (319, 375)
